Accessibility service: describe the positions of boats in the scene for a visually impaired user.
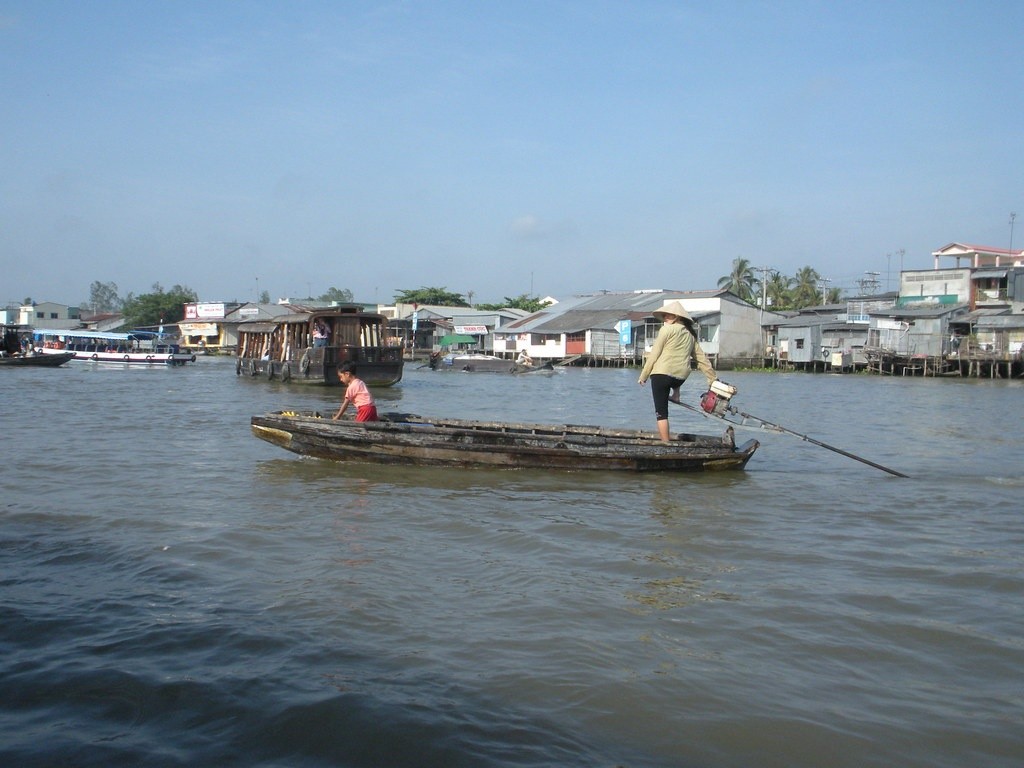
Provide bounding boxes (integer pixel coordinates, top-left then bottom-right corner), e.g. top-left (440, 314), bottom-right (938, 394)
top-left (251, 408), bottom-right (760, 471)
top-left (0, 323), bottom-right (76, 366)
top-left (235, 306), bottom-right (404, 387)
top-left (33, 328), bottom-right (196, 365)
top-left (430, 349), bottom-right (554, 376)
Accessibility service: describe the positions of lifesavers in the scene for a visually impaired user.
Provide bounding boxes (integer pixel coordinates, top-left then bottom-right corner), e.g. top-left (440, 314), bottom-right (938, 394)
top-left (299, 353), bottom-right (310, 373)
top-left (236, 359), bottom-right (241, 375)
top-left (168, 355), bottom-right (174, 361)
top-left (38, 348), bottom-right (43, 353)
top-left (146, 355), bottom-right (151, 361)
top-left (124, 355), bottom-right (129, 360)
top-left (281, 363), bottom-right (289, 381)
top-left (249, 360), bottom-right (256, 376)
top-left (823, 350), bottom-right (830, 357)
top-left (266, 362), bottom-right (273, 380)
top-left (92, 354), bottom-right (98, 359)
top-left (462, 365), bottom-right (470, 373)
top-left (192, 355), bottom-right (196, 362)
top-left (508, 367), bottom-right (514, 373)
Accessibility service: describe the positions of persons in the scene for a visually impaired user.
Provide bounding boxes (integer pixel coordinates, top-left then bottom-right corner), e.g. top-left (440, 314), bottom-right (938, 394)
top-left (332, 359), bottom-right (380, 422)
top-left (20, 334), bottom-right (30, 353)
top-left (312, 319), bottom-right (331, 347)
top-left (518, 349), bottom-right (531, 364)
top-left (68, 338), bottom-right (72, 344)
top-left (637, 301), bottom-right (717, 441)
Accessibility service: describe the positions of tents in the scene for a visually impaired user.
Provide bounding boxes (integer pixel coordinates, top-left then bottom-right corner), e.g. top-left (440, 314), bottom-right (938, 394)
top-left (440, 335), bottom-right (477, 345)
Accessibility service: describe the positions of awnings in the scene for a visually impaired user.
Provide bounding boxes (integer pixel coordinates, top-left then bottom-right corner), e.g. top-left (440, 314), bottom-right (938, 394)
top-left (451, 326), bottom-right (488, 335)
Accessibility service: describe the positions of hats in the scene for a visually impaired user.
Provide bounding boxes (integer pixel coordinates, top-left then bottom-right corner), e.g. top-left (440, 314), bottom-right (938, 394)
top-left (652, 301), bottom-right (695, 325)
